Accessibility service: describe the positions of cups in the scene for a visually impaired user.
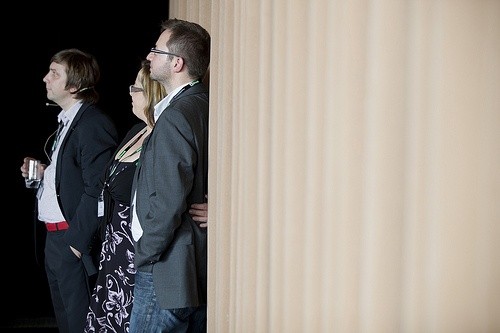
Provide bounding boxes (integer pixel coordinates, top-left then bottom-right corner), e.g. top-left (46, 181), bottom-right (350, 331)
top-left (24, 159), bottom-right (42, 188)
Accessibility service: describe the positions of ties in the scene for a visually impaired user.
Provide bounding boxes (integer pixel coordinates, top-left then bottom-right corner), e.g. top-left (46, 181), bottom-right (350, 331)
top-left (46, 119), bottom-right (64, 167)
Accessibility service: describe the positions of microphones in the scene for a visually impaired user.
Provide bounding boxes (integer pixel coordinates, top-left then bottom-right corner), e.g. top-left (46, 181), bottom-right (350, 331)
top-left (46, 103), bottom-right (58, 106)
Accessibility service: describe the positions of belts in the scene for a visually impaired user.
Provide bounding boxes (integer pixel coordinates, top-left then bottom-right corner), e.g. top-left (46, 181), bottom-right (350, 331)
top-left (45, 220), bottom-right (69, 232)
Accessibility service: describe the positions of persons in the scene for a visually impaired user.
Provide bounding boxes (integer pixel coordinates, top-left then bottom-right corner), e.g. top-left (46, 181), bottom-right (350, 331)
top-left (83, 59), bottom-right (209, 333)
top-left (21, 48), bottom-right (122, 333)
top-left (129, 20), bottom-right (209, 333)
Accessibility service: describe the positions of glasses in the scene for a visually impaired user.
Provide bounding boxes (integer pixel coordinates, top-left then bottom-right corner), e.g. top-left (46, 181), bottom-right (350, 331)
top-left (129, 85), bottom-right (145, 96)
top-left (149, 44), bottom-right (180, 57)
